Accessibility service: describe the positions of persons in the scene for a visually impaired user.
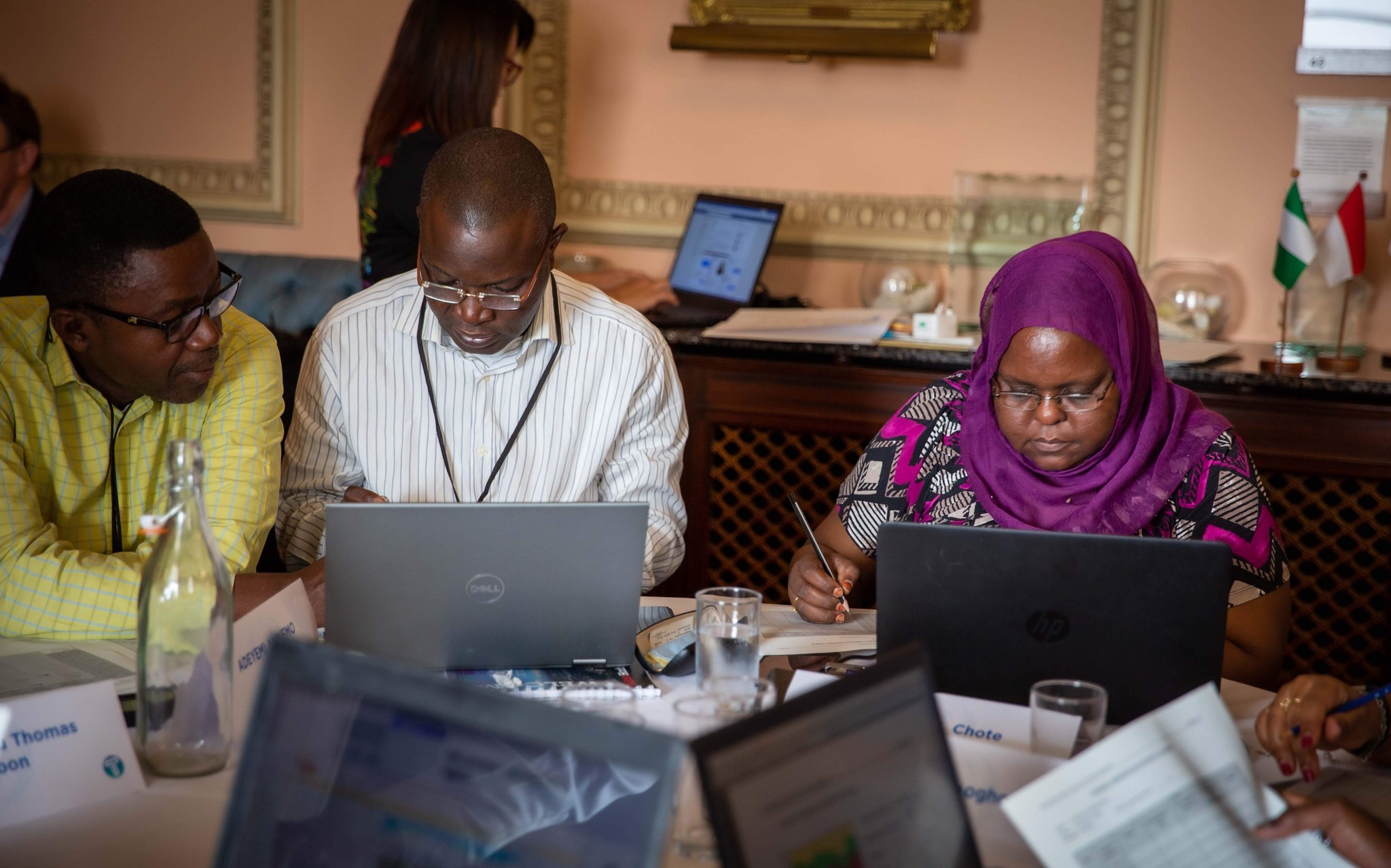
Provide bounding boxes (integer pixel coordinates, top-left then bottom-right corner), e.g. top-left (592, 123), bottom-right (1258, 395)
top-left (276, 127), bottom-right (688, 595)
top-left (0, 169), bottom-right (325, 639)
top-left (787, 232), bottom-right (1299, 685)
top-left (352, 0), bottom-right (536, 291)
top-left (1252, 789), bottom-right (1391, 868)
top-left (1256, 673), bottom-right (1391, 784)
top-left (0, 76), bottom-right (47, 296)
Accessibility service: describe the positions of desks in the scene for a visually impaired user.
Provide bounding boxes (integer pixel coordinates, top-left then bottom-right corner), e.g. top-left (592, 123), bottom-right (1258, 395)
top-left (399, 292), bottom-right (1391, 689)
top-left (0, 594), bottom-right (1391, 868)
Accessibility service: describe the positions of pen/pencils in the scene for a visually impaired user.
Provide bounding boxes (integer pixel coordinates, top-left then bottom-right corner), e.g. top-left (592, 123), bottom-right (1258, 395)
top-left (786, 491), bottom-right (852, 616)
top-left (1282, 684), bottom-right (1391, 742)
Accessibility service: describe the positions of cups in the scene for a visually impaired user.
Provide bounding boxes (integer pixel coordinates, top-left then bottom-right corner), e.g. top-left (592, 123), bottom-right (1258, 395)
top-left (694, 588), bottom-right (764, 734)
top-left (1028, 677), bottom-right (1108, 761)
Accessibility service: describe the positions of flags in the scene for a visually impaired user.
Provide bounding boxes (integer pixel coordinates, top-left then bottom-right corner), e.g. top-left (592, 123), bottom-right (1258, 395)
top-left (1319, 181), bottom-right (1367, 290)
top-left (1273, 180), bottom-right (1320, 292)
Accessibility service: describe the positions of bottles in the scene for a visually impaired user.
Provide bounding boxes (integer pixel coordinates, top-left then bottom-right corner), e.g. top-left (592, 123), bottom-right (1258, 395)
top-left (134, 436), bottom-right (235, 777)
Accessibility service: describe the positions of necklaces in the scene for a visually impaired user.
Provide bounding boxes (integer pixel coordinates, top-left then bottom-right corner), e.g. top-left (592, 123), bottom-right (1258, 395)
top-left (417, 271), bottom-right (563, 503)
top-left (92, 388), bottom-right (134, 554)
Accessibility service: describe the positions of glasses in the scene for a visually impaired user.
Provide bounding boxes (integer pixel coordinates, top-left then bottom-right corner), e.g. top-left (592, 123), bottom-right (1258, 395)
top-left (991, 373), bottom-right (1116, 412)
top-left (501, 56), bottom-right (523, 87)
top-left (416, 229), bottom-right (552, 310)
top-left (45, 259), bottom-right (243, 344)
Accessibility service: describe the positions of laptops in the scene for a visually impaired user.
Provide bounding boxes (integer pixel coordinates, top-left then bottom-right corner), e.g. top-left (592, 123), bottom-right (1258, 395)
top-left (689, 643), bottom-right (984, 868)
top-left (637, 192), bottom-right (784, 328)
top-left (323, 500), bottom-right (650, 672)
top-left (210, 634), bottom-right (685, 868)
top-left (876, 523), bottom-right (1231, 724)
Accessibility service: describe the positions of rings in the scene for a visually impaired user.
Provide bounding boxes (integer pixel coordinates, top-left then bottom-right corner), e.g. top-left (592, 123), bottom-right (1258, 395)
top-left (1279, 697), bottom-right (1301, 710)
top-left (793, 597), bottom-right (799, 613)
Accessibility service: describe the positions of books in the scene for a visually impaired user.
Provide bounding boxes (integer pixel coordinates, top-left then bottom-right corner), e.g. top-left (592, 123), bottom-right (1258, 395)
top-left (0, 640), bottom-right (137, 702)
top-left (636, 603), bottom-right (876, 672)
top-left (510, 669), bottom-right (1359, 868)
